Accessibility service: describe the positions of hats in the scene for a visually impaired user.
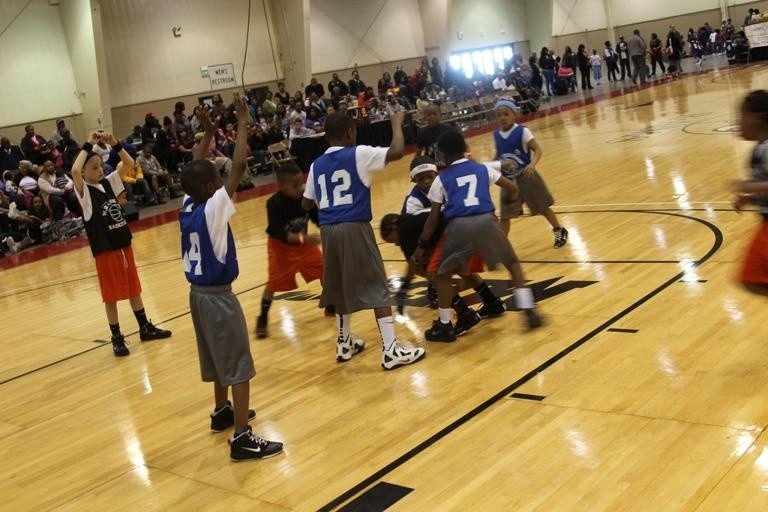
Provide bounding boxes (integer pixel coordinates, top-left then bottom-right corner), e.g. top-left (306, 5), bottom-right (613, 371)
top-left (493, 94), bottom-right (519, 116)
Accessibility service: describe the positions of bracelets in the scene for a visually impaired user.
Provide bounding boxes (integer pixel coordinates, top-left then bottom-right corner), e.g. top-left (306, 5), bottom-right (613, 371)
top-left (82, 142), bottom-right (93, 154)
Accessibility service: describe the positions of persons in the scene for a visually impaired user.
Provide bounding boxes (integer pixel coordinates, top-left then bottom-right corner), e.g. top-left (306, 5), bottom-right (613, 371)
top-left (491, 99), bottom-right (569, 249)
top-left (302, 109), bottom-right (540, 370)
top-left (88, 82), bottom-right (323, 205)
top-left (71, 132), bottom-right (171, 357)
top-left (648, 8), bottom-right (766, 77)
top-left (0, 118), bottom-right (81, 258)
top-left (511, 30), bottom-right (650, 102)
top-left (733, 90), bottom-right (767, 298)
top-left (256, 164), bottom-right (337, 339)
top-left (304, 55), bottom-right (515, 118)
top-left (178, 89), bottom-right (283, 461)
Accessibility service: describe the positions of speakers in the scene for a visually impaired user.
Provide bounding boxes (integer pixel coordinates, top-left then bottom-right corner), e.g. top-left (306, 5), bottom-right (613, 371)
top-left (120, 202), bottom-right (139, 223)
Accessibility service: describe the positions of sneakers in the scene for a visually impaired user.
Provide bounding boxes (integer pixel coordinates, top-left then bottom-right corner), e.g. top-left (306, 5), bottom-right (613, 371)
top-left (137, 320), bottom-right (173, 342)
top-left (423, 307), bottom-right (482, 343)
top-left (524, 308), bottom-right (541, 328)
top-left (379, 337), bottom-right (427, 370)
top-left (552, 226), bottom-right (569, 250)
top-left (253, 315), bottom-right (267, 339)
top-left (205, 400), bottom-right (257, 434)
top-left (7, 201), bottom-right (19, 220)
top-left (109, 333), bottom-right (130, 357)
top-left (229, 425), bottom-right (287, 463)
top-left (140, 190), bottom-right (185, 205)
top-left (476, 299), bottom-right (508, 319)
top-left (333, 334), bottom-right (365, 362)
top-left (426, 282), bottom-right (440, 310)
top-left (6, 235), bottom-right (18, 255)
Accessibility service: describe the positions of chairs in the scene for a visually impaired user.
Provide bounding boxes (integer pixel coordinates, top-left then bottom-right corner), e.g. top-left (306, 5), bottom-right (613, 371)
top-left (39, 60), bottom-right (537, 246)
top-left (740, 47), bottom-right (752, 64)
